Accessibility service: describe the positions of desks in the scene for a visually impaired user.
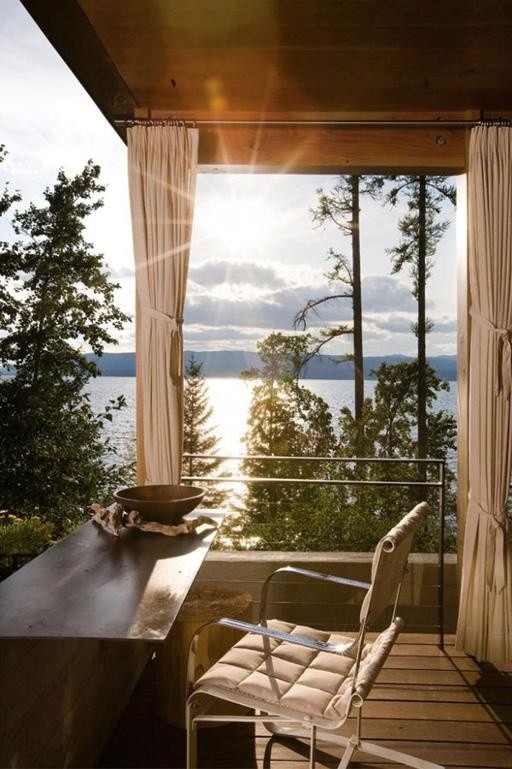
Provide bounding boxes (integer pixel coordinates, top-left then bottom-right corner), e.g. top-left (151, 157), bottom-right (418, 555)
top-left (0, 508), bottom-right (229, 768)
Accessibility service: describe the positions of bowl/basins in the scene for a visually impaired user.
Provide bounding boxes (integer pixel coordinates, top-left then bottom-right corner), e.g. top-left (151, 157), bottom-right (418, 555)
top-left (113, 484), bottom-right (207, 526)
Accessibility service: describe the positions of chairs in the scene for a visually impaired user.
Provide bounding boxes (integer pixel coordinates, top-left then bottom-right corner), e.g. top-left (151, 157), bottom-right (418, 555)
top-left (184, 500), bottom-right (446, 769)
